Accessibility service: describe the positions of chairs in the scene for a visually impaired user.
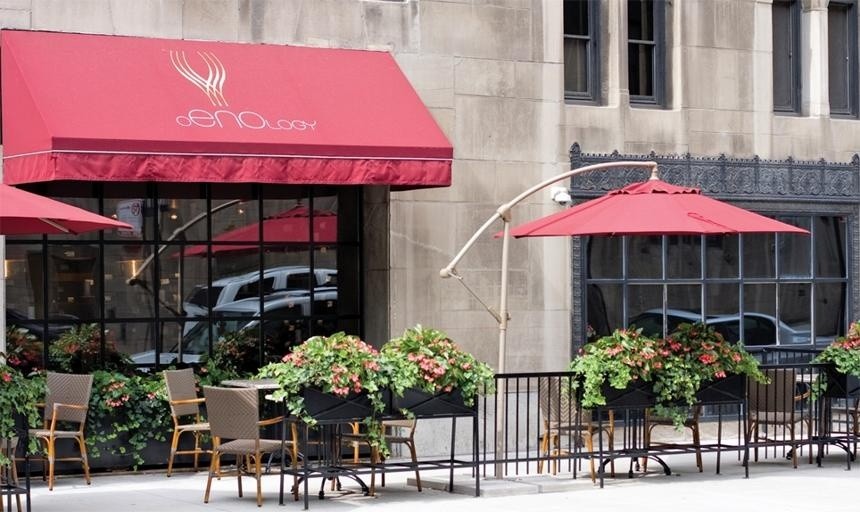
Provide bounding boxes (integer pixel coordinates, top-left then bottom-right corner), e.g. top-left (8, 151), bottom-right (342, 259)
top-left (376, 325), bottom-right (497, 408)
top-left (337, 416), bottom-right (423, 496)
top-left (203, 384), bottom-right (298, 507)
top-left (538, 368), bottom-right (860, 485)
top-left (165, 366), bottom-right (219, 476)
top-left (6, 374), bottom-right (96, 490)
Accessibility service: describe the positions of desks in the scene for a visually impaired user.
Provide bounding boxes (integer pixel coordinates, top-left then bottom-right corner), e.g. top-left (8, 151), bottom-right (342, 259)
top-left (220, 377), bottom-right (385, 497)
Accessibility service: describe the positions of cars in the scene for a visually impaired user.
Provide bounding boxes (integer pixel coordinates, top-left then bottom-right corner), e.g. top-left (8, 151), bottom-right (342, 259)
top-left (626, 303), bottom-right (837, 414)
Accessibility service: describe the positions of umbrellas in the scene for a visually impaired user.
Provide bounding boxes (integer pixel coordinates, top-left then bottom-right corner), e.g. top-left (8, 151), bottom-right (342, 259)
top-left (437, 159), bottom-right (815, 478)
top-left (121, 196), bottom-right (338, 365)
top-left (0, 179), bottom-right (135, 237)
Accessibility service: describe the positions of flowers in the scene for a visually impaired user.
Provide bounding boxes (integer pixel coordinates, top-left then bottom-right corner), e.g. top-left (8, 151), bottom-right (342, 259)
top-left (86, 369), bottom-right (166, 471)
top-left (658, 323), bottom-right (773, 428)
top-left (253, 330), bottom-right (391, 457)
top-left (804, 326), bottom-right (860, 399)
top-left (569, 325), bottom-right (692, 409)
top-left (189, 350), bottom-right (252, 393)
